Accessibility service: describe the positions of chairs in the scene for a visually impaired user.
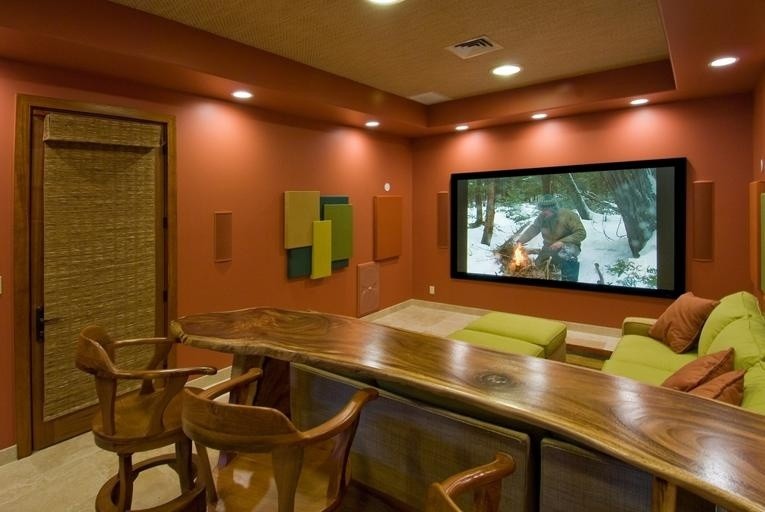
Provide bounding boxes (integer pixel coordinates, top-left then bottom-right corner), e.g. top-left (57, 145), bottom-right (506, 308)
top-left (179, 363), bottom-right (381, 511)
top-left (427, 445), bottom-right (522, 512)
top-left (73, 323), bottom-right (220, 511)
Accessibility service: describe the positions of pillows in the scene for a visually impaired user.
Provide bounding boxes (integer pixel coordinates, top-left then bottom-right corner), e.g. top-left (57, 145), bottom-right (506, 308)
top-left (688, 367), bottom-right (746, 410)
top-left (648, 291), bottom-right (722, 354)
top-left (660, 346), bottom-right (737, 393)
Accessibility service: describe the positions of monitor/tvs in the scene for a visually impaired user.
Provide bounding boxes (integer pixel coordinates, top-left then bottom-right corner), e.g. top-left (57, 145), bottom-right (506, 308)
top-left (450, 156), bottom-right (688, 299)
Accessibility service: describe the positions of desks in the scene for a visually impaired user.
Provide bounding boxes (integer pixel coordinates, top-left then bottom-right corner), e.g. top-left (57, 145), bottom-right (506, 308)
top-left (169, 305), bottom-right (765, 512)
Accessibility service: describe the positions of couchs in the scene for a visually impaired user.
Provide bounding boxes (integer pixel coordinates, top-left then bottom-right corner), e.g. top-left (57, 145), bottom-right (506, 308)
top-left (466, 312), bottom-right (571, 362)
top-left (601, 288), bottom-right (765, 415)
top-left (443, 329), bottom-right (546, 361)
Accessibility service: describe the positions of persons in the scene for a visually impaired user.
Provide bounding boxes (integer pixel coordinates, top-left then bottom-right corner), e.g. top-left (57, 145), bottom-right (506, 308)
top-left (516, 194), bottom-right (586, 282)
